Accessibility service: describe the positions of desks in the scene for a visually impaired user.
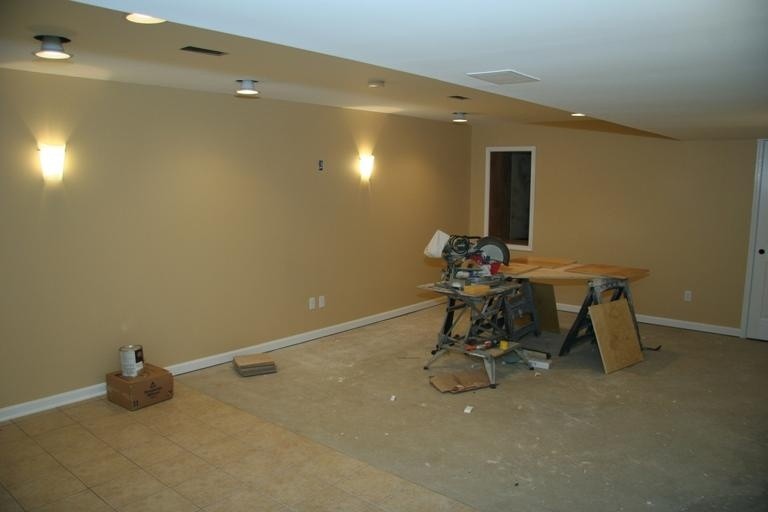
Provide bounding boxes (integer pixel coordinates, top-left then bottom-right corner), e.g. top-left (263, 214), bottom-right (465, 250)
top-left (414, 277), bottom-right (542, 343)
top-left (426, 256), bottom-right (650, 356)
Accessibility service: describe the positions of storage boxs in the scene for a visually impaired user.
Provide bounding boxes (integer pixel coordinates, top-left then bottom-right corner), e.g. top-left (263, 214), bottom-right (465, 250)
top-left (105, 363), bottom-right (174, 412)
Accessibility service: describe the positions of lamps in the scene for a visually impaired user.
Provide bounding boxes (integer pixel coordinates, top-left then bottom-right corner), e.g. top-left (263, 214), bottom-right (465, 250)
top-left (235, 79), bottom-right (261, 97)
top-left (450, 112), bottom-right (468, 123)
top-left (33, 35), bottom-right (72, 60)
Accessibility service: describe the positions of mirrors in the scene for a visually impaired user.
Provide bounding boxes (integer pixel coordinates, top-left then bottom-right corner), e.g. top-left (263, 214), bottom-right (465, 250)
top-left (484, 145), bottom-right (535, 251)
